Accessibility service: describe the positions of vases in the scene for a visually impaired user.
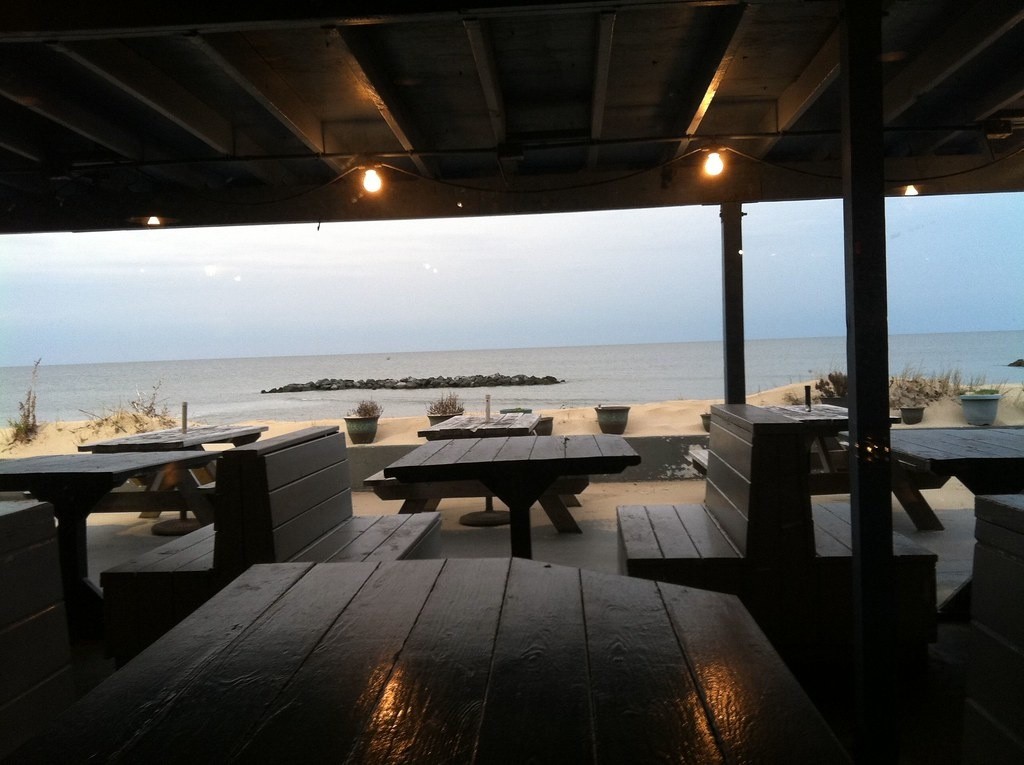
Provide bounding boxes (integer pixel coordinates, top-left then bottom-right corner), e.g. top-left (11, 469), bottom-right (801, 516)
top-left (533, 415), bottom-right (555, 435)
top-left (699, 413), bottom-right (711, 432)
top-left (900, 405), bottom-right (926, 424)
top-left (594, 405), bottom-right (631, 435)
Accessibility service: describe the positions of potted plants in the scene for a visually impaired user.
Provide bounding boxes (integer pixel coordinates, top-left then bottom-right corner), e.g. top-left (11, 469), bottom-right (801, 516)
top-left (958, 388), bottom-right (1002, 426)
top-left (424, 390), bottom-right (465, 426)
top-left (342, 398), bottom-right (385, 444)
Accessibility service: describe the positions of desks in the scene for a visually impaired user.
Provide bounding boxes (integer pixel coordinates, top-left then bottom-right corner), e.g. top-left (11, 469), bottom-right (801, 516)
top-left (0, 450), bottom-right (226, 667)
top-left (758, 403), bottom-right (902, 493)
top-left (417, 413), bottom-right (543, 527)
top-left (839, 428), bottom-right (1024, 498)
top-left (0, 559), bottom-right (861, 765)
top-left (383, 433), bottom-right (641, 561)
top-left (77, 424), bottom-right (270, 537)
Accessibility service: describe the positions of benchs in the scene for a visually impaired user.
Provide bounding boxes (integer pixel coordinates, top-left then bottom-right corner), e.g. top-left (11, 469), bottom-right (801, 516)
top-left (0, 498), bottom-right (132, 763)
top-left (732, 403), bottom-right (940, 646)
top-left (239, 423), bottom-right (445, 575)
top-left (538, 473), bottom-right (589, 534)
top-left (891, 459), bottom-right (952, 531)
top-left (615, 403), bottom-right (784, 639)
top-left (689, 449), bottom-right (710, 474)
top-left (362, 468), bottom-right (443, 514)
top-left (97, 425), bottom-right (314, 660)
